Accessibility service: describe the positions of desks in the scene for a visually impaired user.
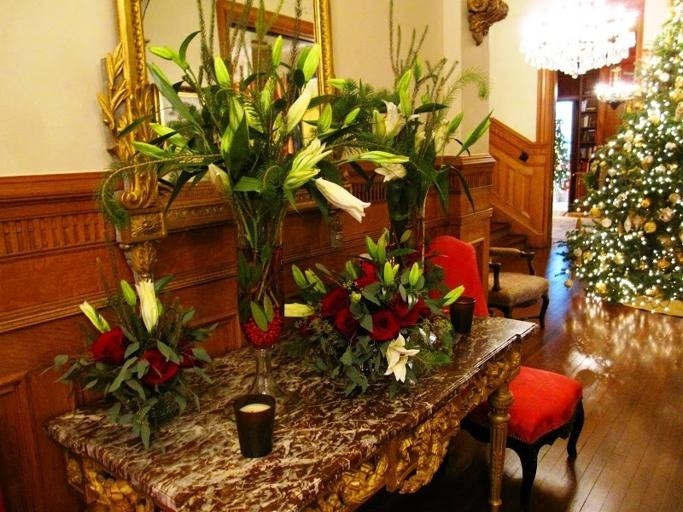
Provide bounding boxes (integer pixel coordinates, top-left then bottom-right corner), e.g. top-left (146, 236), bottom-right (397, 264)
top-left (37, 315), bottom-right (539, 511)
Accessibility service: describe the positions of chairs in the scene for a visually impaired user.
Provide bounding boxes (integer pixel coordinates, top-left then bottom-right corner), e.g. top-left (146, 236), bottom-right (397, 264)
top-left (425, 234), bottom-right (582, 497)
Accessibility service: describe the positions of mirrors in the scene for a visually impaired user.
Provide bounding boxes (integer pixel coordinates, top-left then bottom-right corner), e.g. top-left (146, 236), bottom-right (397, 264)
top-left (94, 1), bottom-right (351, 248)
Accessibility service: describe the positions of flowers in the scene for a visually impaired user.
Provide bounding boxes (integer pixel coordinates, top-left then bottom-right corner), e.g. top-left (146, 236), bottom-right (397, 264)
top-left (41, 271), bottom-right (222, 458)
top-left (488, 246), bottom-right (551, 330)
top-left (94, 1), bottom-right (370, 342)
top-left (336, 0), bottom-right (493, 221)
top-left (302, 232), bottom-right (456, 389)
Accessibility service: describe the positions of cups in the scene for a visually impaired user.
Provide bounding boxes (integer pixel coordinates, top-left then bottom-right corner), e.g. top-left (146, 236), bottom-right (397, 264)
top-left (234, 393), bottom-right (276, 457)
top-left (450, 296), bottom-right (476, 334)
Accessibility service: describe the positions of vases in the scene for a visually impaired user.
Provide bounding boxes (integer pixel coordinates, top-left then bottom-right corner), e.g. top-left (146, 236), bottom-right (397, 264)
top-left (381, 175), bottom-right (430, 251)
top-left (225, 197), bottom-right (292, 410)
